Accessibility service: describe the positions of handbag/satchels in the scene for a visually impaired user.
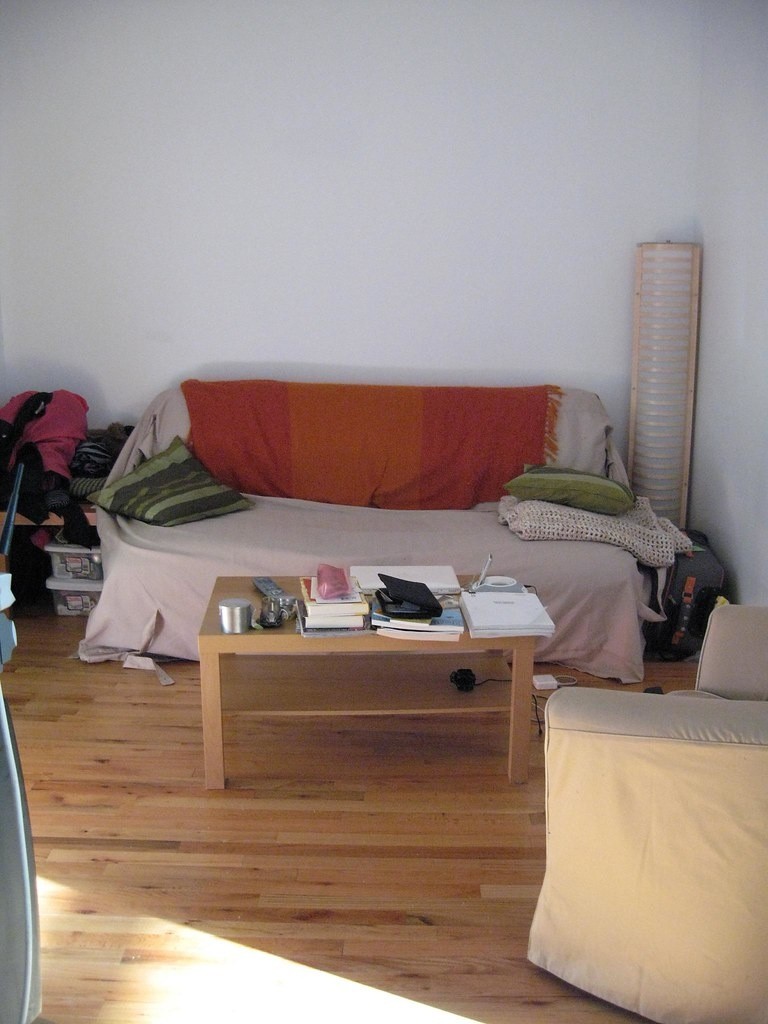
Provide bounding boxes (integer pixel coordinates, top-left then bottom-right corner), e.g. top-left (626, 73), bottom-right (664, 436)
top-left (317, 564), bottom-right (352, 600)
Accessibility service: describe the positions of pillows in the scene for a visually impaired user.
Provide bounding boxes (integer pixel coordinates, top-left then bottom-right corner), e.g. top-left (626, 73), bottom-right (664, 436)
top-left (505, 459), bottom-right (636, 516)
top-left (86, 434), bottom-right (255, 528)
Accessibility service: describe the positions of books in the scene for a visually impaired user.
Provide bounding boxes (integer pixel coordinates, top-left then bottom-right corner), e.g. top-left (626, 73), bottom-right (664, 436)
top-left (299, 575), bottom-right (556, 643)
top-left (349, 565), bottom-right (461, 594)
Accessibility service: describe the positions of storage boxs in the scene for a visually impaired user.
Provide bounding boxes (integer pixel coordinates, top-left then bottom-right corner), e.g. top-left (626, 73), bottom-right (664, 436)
top-left (48, 544), bottom-right (103, 616)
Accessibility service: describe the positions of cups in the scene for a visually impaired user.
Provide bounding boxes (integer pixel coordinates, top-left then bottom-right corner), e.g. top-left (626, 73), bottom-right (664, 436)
top-left (260, 597), bottom-right (289, 628)
top-left (218, 599), bottom-right (251, 634)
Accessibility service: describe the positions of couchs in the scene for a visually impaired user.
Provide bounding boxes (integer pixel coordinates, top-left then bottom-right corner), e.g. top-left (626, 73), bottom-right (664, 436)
top-left (78, 378), bottom-right (652, 685)
top-left (526, 605), bottom-right (768, 1023)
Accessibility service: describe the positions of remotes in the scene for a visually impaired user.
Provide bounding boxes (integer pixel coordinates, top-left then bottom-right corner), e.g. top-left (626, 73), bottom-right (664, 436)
top-left (251, 575), bottom-right (297, 608)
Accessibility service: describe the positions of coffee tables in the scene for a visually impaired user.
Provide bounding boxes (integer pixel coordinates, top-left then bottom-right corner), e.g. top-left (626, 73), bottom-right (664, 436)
top-left (199, 577), bottom-right (541, 792)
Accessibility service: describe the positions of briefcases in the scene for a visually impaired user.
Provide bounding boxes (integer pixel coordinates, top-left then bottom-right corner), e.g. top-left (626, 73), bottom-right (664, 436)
top-left (652, 529), bottom-right (724, 661)
